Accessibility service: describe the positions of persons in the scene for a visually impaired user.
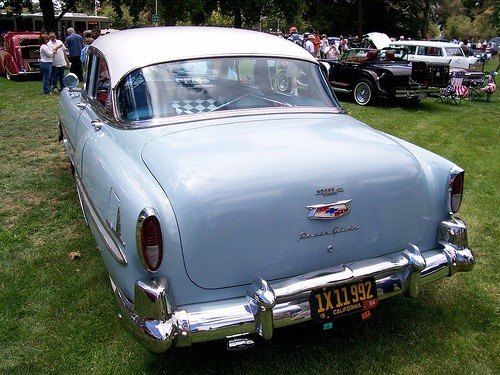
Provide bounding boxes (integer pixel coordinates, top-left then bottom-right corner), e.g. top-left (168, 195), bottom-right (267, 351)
top-left (225, 26), bottom-right (500, 97)
top-left (40, 27), bottom-right (110, 95)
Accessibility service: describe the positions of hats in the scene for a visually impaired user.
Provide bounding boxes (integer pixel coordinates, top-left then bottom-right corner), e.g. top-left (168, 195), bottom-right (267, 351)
top-left (329, 38), bottom-right (336, 43)
top-left (308, 35), bottom-right (314, 39)
top-left (322, 34), bottom-right (327, 37)
top-left (290, 26), bottom-right (298, 32)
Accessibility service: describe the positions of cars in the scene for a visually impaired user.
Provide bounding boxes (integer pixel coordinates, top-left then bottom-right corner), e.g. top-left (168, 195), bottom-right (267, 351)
top-left (58, 25), bottom-right (475, 355)
top-left (276, 48), bottom-right (427, 110)
top-left (0, 31), bottom-right (50, 80)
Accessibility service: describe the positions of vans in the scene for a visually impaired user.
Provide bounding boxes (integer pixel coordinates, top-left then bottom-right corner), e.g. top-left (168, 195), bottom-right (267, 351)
top-left (364, 32), bottom-right (470, 70)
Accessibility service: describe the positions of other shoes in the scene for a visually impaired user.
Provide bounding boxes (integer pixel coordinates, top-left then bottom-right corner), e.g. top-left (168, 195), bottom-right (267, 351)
top-left (44, 92), bottom-right (52, 95)
top-left (54, 88), bottom-right (58, 93)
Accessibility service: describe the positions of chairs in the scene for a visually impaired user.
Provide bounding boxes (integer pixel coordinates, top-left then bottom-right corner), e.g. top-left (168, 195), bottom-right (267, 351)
top-left (434, 70), bottom-right (497, 106)
top-left (362, 51), bottom-right (379, 61)
top-left (384, 52), bottom-right (395, 61)
